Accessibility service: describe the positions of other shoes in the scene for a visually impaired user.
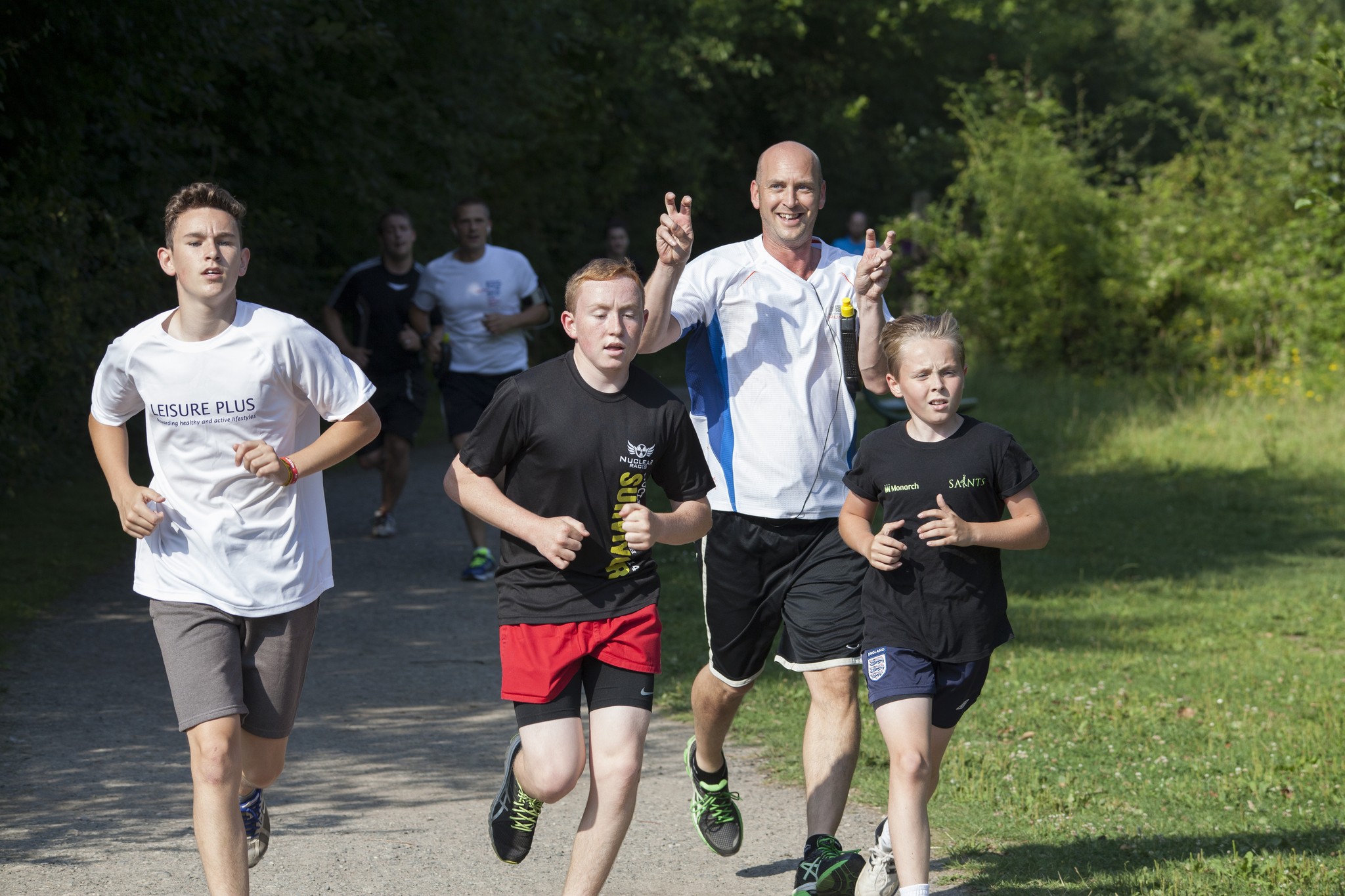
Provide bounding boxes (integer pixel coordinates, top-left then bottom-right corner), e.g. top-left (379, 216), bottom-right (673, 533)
top-left (371, 511), bottom-right (395, 539)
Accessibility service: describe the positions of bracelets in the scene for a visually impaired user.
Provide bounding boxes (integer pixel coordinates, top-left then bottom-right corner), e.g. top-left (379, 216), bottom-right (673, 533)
top-left (279, 456), bottom-right (298, 485)
top-left (278, 458), bottom-right (293, 487)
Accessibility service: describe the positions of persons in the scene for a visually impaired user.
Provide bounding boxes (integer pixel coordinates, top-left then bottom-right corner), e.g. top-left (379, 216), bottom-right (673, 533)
top-left (411, 194), bottom-right (546, 579)
top-left (86, 181), bottom-right (381, 896)
top-left (638, 138), bottom-right (902, 896)
top-left (834, 210), bottom-right (886, 255)
top-left (323, 205), bottom-right (443, 538)
top-left (837, 309), bottom-right (1051, 896)
top-left (442, 257), bottom-right (718, 894)
top-left (604, 225), bottom-right (644, 274)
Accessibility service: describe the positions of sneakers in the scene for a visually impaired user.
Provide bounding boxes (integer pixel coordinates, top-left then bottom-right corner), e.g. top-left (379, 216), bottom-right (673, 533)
top-left (855, 814), bottom-right (900, 896)
top-left (463, 545), bottom-right (499, 581)
top-left (792, 849), bottom-right (867, 896)
top-left (683, 735), bottom-right (743, 857)
top-left (239, 788), bottom-right (271, 869)
top-left (488, 733), bottom-right (546, 866)
